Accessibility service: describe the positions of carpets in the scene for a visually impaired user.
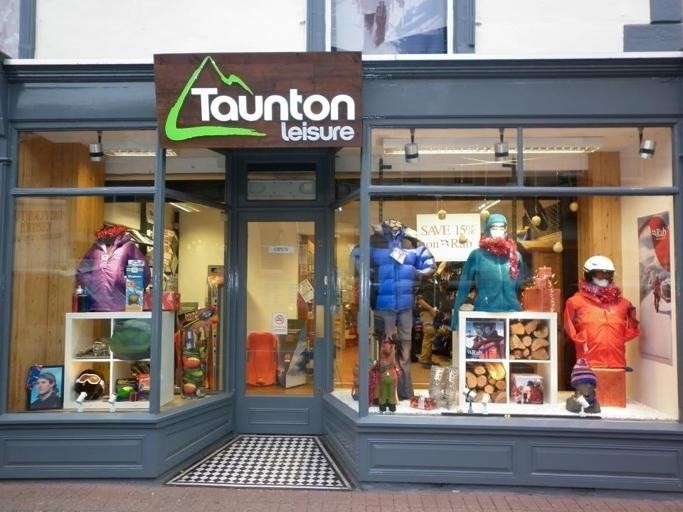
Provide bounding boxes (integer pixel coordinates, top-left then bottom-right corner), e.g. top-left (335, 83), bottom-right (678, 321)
top-left (160, 432), bottom-right (357, 493)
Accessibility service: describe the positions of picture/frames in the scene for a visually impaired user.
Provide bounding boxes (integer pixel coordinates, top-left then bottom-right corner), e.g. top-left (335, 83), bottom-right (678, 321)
top-left (25, 364), bottom-right (63, 414)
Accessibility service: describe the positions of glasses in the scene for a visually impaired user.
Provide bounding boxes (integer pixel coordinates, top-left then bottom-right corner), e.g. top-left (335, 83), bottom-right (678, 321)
top-left (489, 225), bottom-right (505, 230)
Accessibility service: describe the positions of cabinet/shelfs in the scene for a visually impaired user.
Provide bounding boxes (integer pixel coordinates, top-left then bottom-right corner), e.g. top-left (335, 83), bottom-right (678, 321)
top-left (59, 305), bottom-right (176, 410)
top-left (451, 308), bottom-right (562, 412)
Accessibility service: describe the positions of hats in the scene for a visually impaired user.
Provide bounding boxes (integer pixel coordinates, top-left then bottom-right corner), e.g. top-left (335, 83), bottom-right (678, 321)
top-left (570, 357), bottom-right (599, 388)
top-left (527, 381), bottom-right (535, 386)
top-left (487, 213), bottom-right (507, 227)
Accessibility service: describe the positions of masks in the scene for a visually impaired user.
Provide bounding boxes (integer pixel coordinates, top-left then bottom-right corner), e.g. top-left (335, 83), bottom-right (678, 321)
top-left (592, 276), bottom-right (617, 288)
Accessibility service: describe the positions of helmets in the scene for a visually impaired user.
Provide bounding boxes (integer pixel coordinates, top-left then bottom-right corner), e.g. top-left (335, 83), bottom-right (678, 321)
top-left (473, 323), bottom-right (496, 338)
top-left (582, 256), bottom-right (615, 274)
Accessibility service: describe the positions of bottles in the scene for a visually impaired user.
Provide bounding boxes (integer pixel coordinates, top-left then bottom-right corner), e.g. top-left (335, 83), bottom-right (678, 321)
top-left (198, 329), bottom-right (206, 355)
top-left (69, 285), bottom-right (89, 311)
top-left (185, 331), bottom-right (194, 350)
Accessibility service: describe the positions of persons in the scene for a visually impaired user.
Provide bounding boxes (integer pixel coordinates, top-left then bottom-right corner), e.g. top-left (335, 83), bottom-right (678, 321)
top-left (30, 372), bottom-right (62, 409)
top-left (567, 360), bottom-right (601, 413)
top-left (564, 255), bottom-right (642, 369)
top-left (653, 275), bottom-right (662, 314)
top-left (351, 219), bottom-right (436, 399)
top-left (76, 223), bottom-right (151, 310)
top-left (449, 213), bottom-right (525, 330)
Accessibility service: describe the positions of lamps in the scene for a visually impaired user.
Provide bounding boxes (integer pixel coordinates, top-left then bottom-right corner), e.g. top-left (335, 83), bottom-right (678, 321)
top-left (87, 132), bottom-right (104, 163)
top-left (492, 128), bottom-right (511, 163)
top-left (403, 128), bottom-right (418, 166)
top-left (635, 126), bottom-right (657, 160)
